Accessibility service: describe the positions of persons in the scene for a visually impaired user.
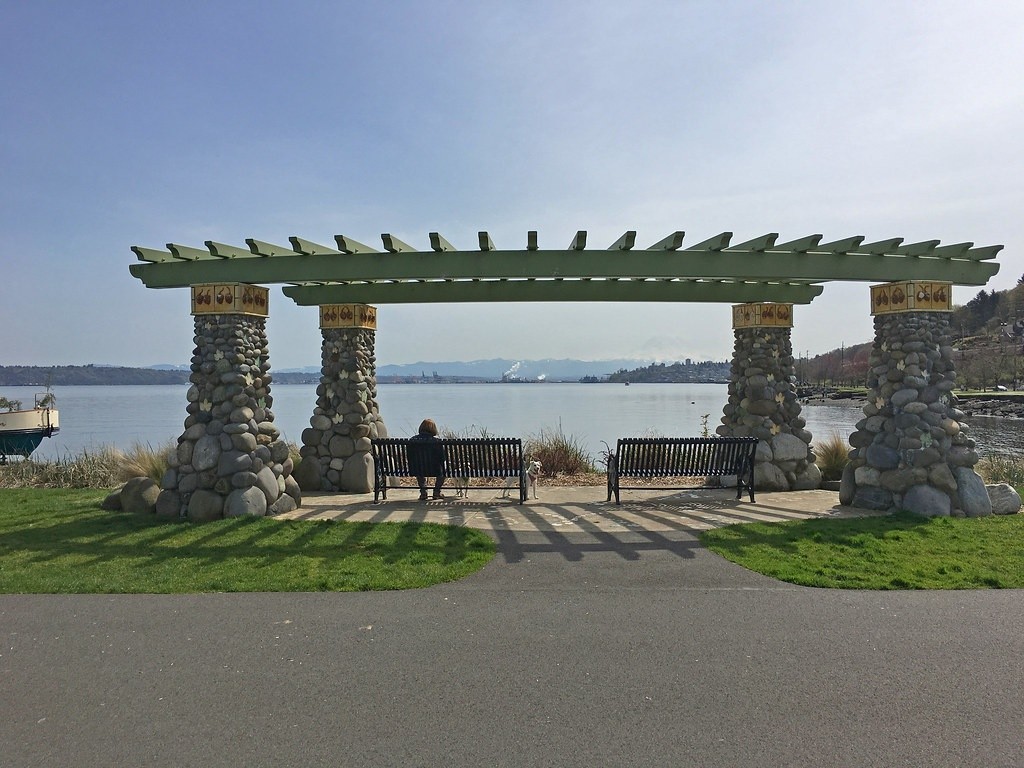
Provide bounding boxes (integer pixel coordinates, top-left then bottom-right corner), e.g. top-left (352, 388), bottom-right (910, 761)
top-left (406, 418), bottom-right (449, 500)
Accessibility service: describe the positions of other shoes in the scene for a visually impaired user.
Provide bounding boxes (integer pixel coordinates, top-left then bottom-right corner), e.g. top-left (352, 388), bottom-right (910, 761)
top-left (433, 493), bottom-right (444, 499)
top-left (420, 493), bottom-right (427, 499)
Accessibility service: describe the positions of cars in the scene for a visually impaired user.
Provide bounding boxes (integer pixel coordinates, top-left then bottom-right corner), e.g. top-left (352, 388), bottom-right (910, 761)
top-left (994, 386), bottom-right (1008, 391)
top-left (1016, 384), bottom-right (1024, 391)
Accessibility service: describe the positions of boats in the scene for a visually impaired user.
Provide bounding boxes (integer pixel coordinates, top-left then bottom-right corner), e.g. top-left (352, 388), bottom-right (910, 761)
top-left (0, 390), bottom-right (60, 466)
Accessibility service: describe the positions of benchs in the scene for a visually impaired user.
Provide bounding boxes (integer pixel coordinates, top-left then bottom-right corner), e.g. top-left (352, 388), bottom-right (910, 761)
top-left (370, 437), bottom-right (527, 505)
top-left (606, 435), bottom-right (759, 504)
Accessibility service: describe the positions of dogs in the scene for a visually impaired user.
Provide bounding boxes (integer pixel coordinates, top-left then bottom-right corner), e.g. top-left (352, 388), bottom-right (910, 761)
top-left (500, 461), bottom-right (542, 500)
top-left (450, 462), bottom-right (470, 498)
